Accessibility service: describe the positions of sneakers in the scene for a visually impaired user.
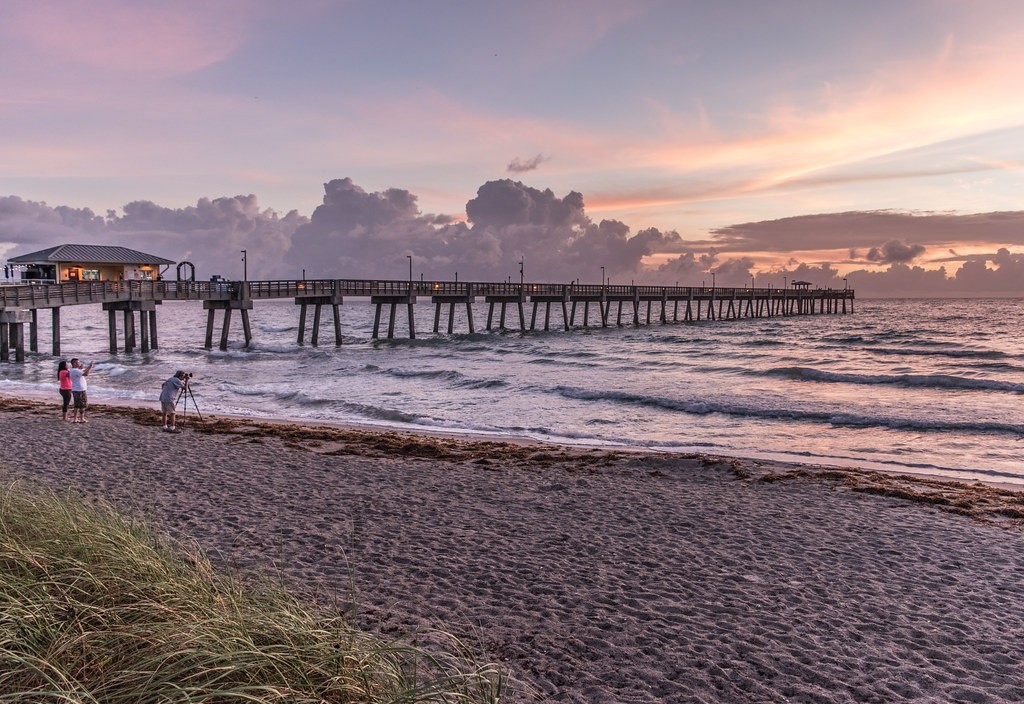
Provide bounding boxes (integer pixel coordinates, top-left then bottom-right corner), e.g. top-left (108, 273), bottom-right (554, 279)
top-left (162, 426), bottom-right (181, 433)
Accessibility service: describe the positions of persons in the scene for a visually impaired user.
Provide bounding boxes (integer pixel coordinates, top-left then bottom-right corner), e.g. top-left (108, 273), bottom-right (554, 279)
top-left (156, 274), bottom-right (163, 292)
top-left (70, 358), bottom-right (92, 424)
top-left (159, 370), bottom-right (190, 434)
top-left (119, 271), bottom-right (124, 291)
top-left (57, 361), bottom-right (83, 422)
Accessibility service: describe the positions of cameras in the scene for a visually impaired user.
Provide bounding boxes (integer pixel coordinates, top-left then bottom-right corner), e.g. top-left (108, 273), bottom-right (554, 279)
top-left (184, 373), bottom-right (193, 378)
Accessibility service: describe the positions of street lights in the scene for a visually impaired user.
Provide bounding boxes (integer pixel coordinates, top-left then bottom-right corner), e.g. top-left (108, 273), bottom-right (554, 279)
top-left (711, 273), bottom-right (715, 288)
top-left (407, 255), bottom-right (412, 282)
top-left (601, 266), bottom-right (604, 285)
top-left (843, 279), bottom-right (847, 289)
top-left (751, 274), bottom-right (755, 289)
top-left (519, 255), bottom-right (524, 284)
top-left (240, 249), bottom-right (247, 281)
top-left (784, 276), bottom-right (787, 289)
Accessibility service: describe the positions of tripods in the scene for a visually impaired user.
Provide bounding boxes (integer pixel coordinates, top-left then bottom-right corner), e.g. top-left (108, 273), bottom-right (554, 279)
top-left (176, 377), bottom-right (206, 435)
